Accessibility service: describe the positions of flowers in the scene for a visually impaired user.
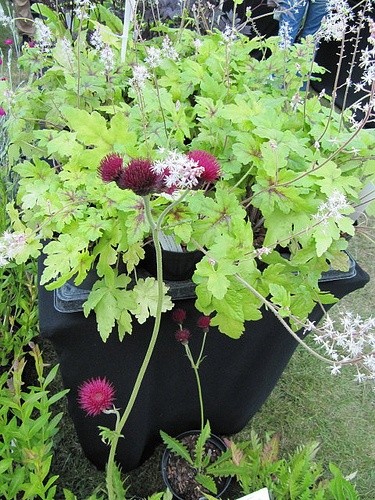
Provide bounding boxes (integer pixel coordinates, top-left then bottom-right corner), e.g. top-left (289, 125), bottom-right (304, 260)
top-left (14, 24), bottom-right (360, 289)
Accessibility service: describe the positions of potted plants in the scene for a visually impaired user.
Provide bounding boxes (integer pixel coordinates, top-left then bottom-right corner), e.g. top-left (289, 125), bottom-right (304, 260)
top-left (28, 228), bottom-right (368, 478)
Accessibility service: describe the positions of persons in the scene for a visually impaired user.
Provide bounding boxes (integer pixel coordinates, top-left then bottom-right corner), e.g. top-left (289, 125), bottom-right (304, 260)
top-left (275, 0), bottom-right (329, 103)
top-left (14, 0), bottom-right (46, 44)
